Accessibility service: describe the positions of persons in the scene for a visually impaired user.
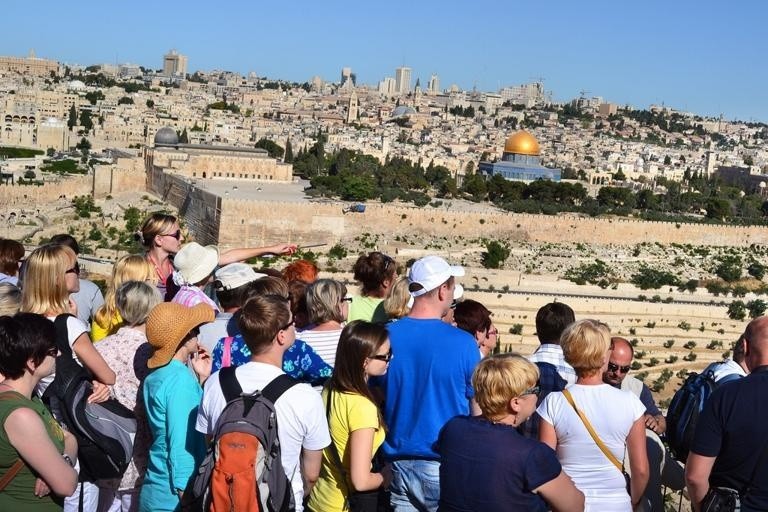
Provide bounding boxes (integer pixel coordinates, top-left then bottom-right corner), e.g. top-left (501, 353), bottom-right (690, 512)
top-left (344, 251), bottom-right (398, 322)
top-left (478, 317), bottom-right (498, 359)
top-left (195, 294), bottom-right (333, 512)
top-left (188, 262), bottom-right (269, 382)
top-left (431, 353), bottom-right (586, 512)
top-left (138, 301), bottom-right (216, 511)
top-left (517, 298), bottom-right (578, 441)
top-left (1, 281), bottom-right (22, 317)
top-left (453, 299), bottom-right (491, 348)
top-left (536, 319), bottom-right (651, 512)
top-left (0, 238), bottom-right (26, 285)
top-left (2, 243), bottom-right (117, 512)
top-left (89, 254), bottom-right (159, 345)
top-left (683, 314), bottom-right (767, 512)
top-left (366, 255), bottom-right (482, 511)
top-left (93, 280), bottom-right (163, 512)
top-left (1, 312), bottom-right (79, 511)
top-left (47, 234), bottom-right (104, 323)
top-left (305, 319), bottom-right (393, 511)
top-left (170, 240), bottom-right (221, 314)
top-left (603, 336), bottom-right (666, 512)
top-left (443, 284), bottom-right (465, 323)
top-left (133, 214), bottom-right (297, 302)
top-left (296, 279), bottom-right (352, 368)
top-left (280, 261), bottom-right (321, 283)
top-left (371, 275), bottom-right (411, 327)
top-left (699, 332), bottom-right (751, 390)
top-left (211, 276), bottom-right (334, 380)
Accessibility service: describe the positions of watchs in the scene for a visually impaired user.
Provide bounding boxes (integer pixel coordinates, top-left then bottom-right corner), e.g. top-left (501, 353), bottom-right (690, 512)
top-left (62, 454), bottom-right (75, 468)
top-left (653, 414), bottom-right (660, 427)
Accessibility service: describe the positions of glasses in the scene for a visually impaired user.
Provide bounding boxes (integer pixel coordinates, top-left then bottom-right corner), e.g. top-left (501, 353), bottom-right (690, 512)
top-left (269, 314), bottom-right (296, 341)
top-left (608, 361), bottom-right (631, 374)
top-left (156, 231), bottom-right (183, 239)
top-left (380, 254), bottom-right (393, 273)
top-left (364, 347), bottom-right (393, 361)
top-left (37, 345), bottom-right (58, 359)
top-left (16, 257), bottom-right (26, 264)
top-left (62, 262), bottom-right (80, 276)
top-left (514, 380), bottom-right (543, 398)
top-left (484, 329), bottom-right (498, 335)
top-left (342, 297), bottom-right (353, 304)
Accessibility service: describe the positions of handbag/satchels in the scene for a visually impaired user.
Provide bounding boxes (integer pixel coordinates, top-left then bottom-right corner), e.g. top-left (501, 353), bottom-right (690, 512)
top-left (699, 487), bottom-right (740, 508)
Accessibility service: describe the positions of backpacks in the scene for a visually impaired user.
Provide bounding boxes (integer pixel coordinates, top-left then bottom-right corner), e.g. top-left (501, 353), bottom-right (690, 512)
top-left (41, 313), bottom-right (138, 483)
top-left (664, 360), bottom-right (746, 464)
top-left (191, 364), bottom-right (297, 512)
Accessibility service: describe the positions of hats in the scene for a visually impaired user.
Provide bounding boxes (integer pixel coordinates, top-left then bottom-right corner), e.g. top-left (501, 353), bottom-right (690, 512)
top-left (173, 242), bottom-right (221, 286)
top-left (215, 263), bottom-right (268, 291)
top-left (450, 284), bottom-right (463, 298)
top-left (145, 301), bottom-right (216, 368)
top-left (407, 256), bottom-right (464, 308)
